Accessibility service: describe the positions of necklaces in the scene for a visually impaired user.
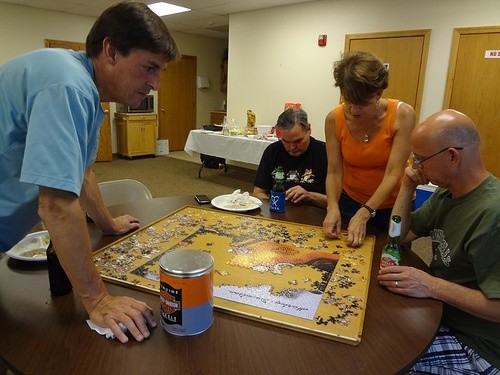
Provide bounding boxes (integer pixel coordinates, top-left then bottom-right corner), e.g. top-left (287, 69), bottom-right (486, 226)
top-left (364, 135), bottom-right (368, 142)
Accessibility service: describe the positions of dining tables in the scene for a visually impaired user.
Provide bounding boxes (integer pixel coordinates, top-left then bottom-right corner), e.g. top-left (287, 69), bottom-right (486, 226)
top-left (0, 194), bottom-right (443, 375)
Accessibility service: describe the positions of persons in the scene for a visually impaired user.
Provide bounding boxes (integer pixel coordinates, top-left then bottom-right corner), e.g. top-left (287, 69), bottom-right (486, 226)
top-left (377, 109), bottom-right (500, 375)
top-left (322, 52), bottom-right (417, 247)
top-left (253, 108), bottom-right (328, 209)
top-left (0, 1), bottom-right (179, 343)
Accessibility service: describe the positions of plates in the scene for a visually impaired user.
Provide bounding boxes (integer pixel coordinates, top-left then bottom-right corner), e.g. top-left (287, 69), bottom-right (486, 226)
top-left (211, 194), bottom-right (262, 213)
top-left (6, 229), bottom-right (50, 261)
top-left (203, 125), bottom-right (223, 132)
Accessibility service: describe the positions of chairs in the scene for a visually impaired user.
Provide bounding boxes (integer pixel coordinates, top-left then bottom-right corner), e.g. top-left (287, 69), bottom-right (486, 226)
top-left (97, 179), bottom-right (152, 205)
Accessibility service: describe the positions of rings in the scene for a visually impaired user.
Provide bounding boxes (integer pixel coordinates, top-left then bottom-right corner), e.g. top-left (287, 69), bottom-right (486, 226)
top-left (395, 281), bottom-right (398, 287)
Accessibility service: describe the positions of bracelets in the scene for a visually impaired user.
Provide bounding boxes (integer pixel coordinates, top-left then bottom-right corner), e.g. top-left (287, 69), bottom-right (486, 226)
top-left (363, 205), bottom-right (376, 217)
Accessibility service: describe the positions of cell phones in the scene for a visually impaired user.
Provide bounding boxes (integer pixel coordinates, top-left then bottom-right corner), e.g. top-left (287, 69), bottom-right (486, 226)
top-left (194, 195), bottom-right (211, 204)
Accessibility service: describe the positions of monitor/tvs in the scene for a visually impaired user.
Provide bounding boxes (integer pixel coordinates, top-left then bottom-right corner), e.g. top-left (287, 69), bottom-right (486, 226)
top-left (117, 94), bottom-right (154, 113)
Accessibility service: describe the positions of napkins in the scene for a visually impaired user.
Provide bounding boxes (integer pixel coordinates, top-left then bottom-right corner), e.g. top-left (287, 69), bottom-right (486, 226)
top-left (85, 319), bottom-right (130, 339)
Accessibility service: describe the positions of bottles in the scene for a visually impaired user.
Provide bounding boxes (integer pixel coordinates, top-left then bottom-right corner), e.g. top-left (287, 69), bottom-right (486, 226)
top-left (380, 215), bottom-right (402, 270)
top-left (46, 240), bottom-right (73, 296)
top-left (270, 165), bottom-right (286, 214)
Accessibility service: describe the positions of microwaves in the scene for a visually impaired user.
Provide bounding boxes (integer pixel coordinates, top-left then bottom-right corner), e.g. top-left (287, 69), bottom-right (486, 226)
top-left (116, 94), bottom-right (155, 113)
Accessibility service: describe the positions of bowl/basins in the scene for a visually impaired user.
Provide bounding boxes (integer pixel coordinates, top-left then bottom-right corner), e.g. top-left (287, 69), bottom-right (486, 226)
top-left (247, 135), bottom-right (256, 138)
top-left (257, 125), bottom-right (273, 135)
top-left (267, 137), bottom-right (278, 141)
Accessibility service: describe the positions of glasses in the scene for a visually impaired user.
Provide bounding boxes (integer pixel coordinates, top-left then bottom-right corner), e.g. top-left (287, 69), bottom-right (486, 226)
top-left (412, 146), bottom-right (463, 170)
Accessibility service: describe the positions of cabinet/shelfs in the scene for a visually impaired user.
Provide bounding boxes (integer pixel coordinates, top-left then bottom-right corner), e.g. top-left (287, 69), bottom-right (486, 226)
top-left (114, 112), bottom-right (158, 158)
top-left (46, 39), bottom-right (113, 162)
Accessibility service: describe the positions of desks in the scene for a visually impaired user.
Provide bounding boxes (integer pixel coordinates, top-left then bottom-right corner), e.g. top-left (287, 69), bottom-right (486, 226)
top-left (184, 128), bottom-right (279, 178)
